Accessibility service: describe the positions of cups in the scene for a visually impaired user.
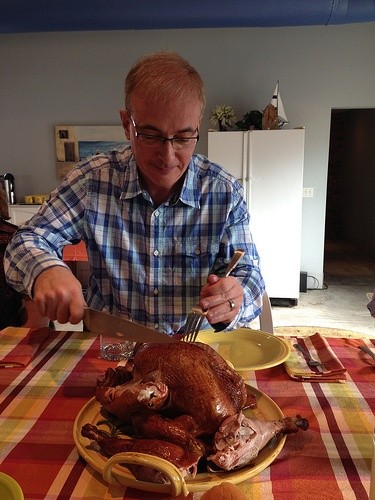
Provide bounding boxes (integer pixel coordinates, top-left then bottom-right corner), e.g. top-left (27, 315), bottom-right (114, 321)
top-left (25, 194), bottom-right (50, 204)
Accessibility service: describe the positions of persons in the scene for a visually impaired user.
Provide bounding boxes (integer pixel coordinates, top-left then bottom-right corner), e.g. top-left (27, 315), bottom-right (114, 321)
top-left (0, 186), bottom-right (55, 330)
top-left (4, 54), bottom-right (265, 334)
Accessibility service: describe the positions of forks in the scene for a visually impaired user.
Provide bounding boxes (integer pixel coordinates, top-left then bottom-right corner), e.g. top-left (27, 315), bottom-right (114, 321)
top-left (185, 251), bottom-right (244, 343)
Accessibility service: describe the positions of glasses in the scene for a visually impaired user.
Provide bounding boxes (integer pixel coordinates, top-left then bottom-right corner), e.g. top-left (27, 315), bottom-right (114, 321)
top-left (128, 112), bottom-right (199, 150)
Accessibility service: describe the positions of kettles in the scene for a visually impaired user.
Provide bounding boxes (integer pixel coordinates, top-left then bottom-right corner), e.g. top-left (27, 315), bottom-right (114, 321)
top-left (0, 173), bottom-right (16, 205)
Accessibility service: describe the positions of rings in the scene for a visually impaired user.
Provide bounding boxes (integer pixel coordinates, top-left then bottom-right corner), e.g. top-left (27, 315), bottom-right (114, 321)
top-left (228, 299), bottom-right (235, 311)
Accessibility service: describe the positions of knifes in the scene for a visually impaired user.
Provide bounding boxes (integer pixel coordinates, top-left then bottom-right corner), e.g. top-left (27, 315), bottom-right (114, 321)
top-left (81, 305), bottom-right (179, 343)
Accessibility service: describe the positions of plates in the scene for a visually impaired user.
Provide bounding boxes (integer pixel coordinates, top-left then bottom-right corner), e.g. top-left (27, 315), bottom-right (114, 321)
top-left (72, 361), bottom-right (287, 495)
top-left (180, 328), bottom-right (291, 371)
top-left (0, 472), bottom-right (24, 500)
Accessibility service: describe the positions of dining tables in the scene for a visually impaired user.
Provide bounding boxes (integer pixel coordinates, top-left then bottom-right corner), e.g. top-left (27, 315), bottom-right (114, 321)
top-left (0, 326), bottom-right (375, 500)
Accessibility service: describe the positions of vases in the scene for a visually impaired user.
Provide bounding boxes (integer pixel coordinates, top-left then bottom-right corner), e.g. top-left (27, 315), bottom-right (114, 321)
top-left (218, 120), bottom-right (227, 131)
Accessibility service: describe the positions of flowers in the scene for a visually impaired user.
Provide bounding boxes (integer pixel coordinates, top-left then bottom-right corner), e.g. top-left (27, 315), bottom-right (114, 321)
top-left (208, 104), bottom-right (237, 127)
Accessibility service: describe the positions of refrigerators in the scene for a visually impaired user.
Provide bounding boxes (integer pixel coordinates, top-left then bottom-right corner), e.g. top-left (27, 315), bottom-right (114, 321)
top-left (208, 126), bottom-right (306, 308)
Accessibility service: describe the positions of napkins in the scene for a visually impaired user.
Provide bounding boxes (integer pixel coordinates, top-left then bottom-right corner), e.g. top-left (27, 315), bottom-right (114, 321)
top-left (276, 332), bottom-right (348, 383)
